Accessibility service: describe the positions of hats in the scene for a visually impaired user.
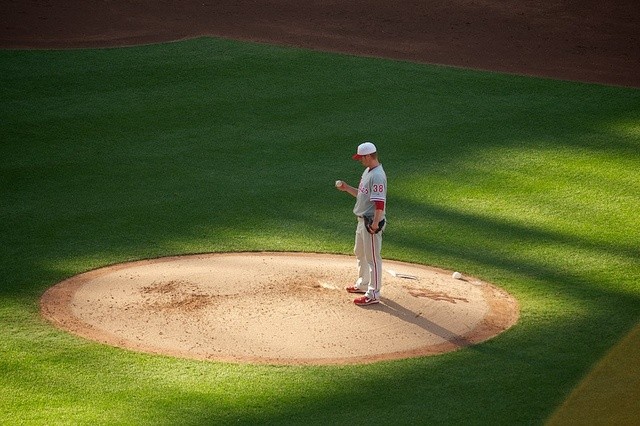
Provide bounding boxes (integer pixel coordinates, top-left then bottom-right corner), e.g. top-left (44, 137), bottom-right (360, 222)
top-left (352, 142), bottom-right (377, 160)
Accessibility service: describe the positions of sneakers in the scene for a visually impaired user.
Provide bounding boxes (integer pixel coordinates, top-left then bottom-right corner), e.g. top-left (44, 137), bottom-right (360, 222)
top-left (345, 285), bottom-right (367, 293)
top-left (354, 296), bottom-right (379, 306)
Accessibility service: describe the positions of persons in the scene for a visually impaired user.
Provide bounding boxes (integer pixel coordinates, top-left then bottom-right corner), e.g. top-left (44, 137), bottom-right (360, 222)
top-left (335, 142), bottom-right (387, 306)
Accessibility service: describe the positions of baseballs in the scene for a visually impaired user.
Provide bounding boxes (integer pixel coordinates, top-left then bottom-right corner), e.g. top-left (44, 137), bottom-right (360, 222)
top-left (335, 180), bottom-right (343, 188)
top-left (452, 272), bottom-right (462, 280)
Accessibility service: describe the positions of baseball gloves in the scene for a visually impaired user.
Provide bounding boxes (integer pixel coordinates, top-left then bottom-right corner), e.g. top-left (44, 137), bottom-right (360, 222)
top-left (363, 214), bottom-right (385, 234)
